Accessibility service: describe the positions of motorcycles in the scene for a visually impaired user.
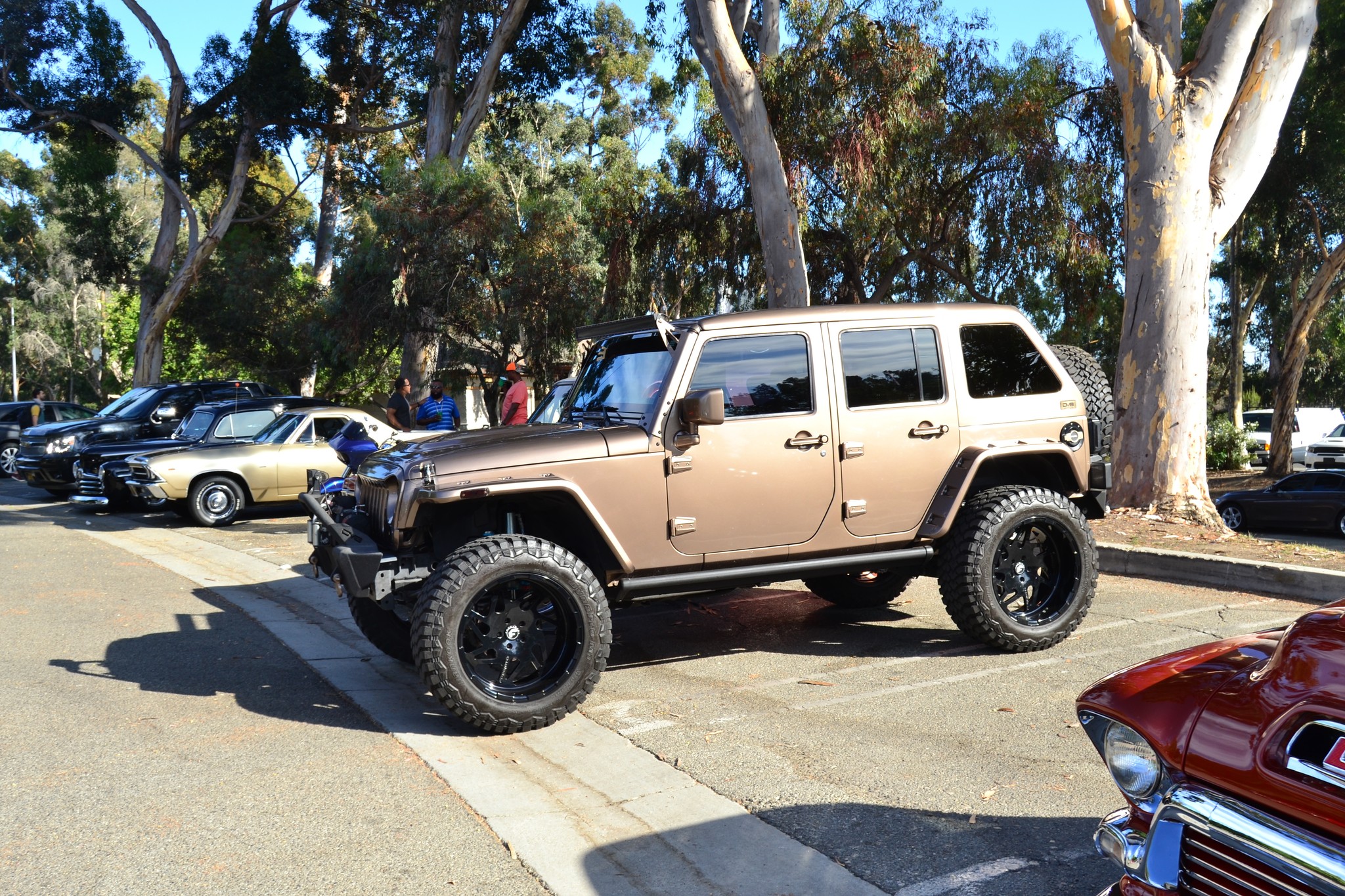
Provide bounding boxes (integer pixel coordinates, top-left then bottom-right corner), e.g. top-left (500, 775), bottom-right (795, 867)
top-left (316, 419), bottom-right (404, 502)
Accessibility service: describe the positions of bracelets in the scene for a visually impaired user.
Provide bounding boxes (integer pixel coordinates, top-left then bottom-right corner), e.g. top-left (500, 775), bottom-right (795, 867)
top-left (417, 402), bottom-right (420, 407)
top-left (501, 421), bottom-right (506, 425)
top-left (456, 427), bottom-right (460, 431)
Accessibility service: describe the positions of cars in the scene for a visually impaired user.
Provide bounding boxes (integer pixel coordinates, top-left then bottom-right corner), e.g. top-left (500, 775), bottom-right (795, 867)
top-left (1216, 468), bottom-right (1345, 539)
top-left (0, 401), bottom-right (98, 479)
top-left (125, 406), bottom-right (455, 529)
top-left (1074, 598), bottom-right (1345, 896)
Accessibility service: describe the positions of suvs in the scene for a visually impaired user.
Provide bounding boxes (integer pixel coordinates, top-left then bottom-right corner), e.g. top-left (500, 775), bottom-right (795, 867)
top-left (12, 377), bottom-right (346, 514)
top-left (297, 304), bottom-right (1112, 736)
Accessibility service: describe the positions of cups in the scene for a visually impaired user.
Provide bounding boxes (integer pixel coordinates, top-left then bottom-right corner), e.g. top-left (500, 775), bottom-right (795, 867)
top-left (437, 412), bottom-right (442, 420)
top-left (498, 376), bottom-right (507, 387)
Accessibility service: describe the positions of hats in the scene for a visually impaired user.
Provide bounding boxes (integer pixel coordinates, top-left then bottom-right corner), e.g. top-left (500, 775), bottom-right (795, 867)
top-left (506, 362), bottom-right (525, 373)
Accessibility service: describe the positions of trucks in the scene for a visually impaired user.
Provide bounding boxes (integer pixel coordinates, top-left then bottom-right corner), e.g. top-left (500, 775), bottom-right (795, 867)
top-left (1242, 408), bottom-right (1345, 470)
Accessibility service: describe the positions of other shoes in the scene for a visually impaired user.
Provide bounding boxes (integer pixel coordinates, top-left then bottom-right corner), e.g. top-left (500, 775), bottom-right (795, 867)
top-left (11, 474), bottom-right (26, 482)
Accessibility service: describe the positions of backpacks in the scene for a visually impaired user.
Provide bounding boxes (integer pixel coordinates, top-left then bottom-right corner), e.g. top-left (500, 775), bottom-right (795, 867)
top-left (18, 403), bottom-right (42, 430)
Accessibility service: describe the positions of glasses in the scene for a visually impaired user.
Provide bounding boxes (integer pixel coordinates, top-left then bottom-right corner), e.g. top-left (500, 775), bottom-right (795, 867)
top-left (430, 385), bottom-right (441, 389)
top-left (402, 383), bottom-right (411, 388)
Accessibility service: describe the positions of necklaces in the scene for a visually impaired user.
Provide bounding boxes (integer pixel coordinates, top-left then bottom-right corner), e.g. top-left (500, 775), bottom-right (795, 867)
top-left (515, 379), bottom-right (521, 383)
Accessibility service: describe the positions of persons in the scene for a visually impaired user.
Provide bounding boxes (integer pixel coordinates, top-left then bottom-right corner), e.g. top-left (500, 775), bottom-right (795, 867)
top-left (499, 380), bottom-right (512, 414)
top-left (386, 377), bottom-right (429, 433)
top-left (500, 362), bottom-right (529, 426)
top-left (417, 379), bottom-right (460, 432)
top-left (12, 389), bottom-right (46, 481)
